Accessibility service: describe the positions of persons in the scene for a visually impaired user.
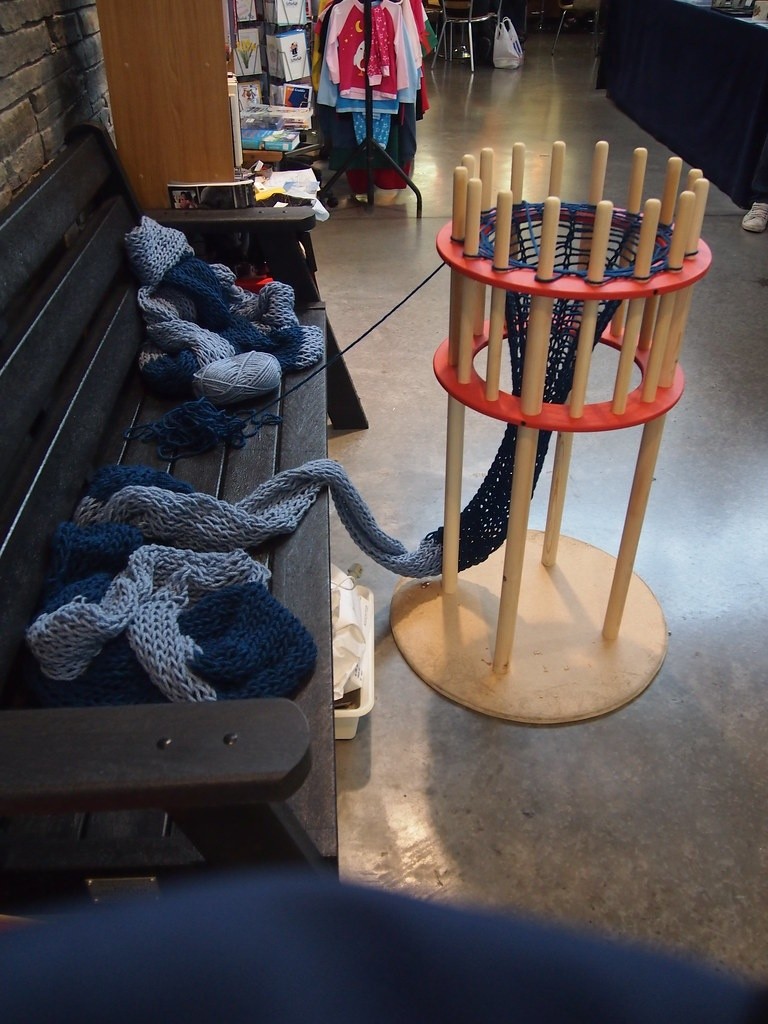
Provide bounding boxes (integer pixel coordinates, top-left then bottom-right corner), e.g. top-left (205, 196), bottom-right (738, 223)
top-left (742, 134), bottom-right (768, 233)
top-left (179, 192), bottom-right (197, 208)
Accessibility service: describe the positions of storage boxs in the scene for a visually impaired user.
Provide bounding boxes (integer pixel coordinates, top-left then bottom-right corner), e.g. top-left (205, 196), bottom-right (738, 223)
top-left (328, 585), bottom-right (376, 741)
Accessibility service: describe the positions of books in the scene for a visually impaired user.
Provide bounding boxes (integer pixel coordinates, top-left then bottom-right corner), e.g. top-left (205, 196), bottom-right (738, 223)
top-left (227, 71), bottom-right (313, 166)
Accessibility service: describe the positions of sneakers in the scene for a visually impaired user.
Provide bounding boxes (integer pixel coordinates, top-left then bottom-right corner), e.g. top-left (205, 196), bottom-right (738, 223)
top-left (742, 202), bottom-right (768, 233)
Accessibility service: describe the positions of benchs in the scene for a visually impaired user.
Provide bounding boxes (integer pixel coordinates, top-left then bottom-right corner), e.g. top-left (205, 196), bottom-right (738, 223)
top-left (2, 121), bottom-right (370, 915)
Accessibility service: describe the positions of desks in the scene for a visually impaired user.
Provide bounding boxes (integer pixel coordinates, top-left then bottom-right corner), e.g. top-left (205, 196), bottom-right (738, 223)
top-left (594, 0), bottom-right (768, 210)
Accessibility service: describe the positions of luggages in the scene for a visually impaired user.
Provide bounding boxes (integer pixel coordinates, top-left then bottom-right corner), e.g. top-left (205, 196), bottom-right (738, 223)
top-left (474, 31), bottom-right (495, 70)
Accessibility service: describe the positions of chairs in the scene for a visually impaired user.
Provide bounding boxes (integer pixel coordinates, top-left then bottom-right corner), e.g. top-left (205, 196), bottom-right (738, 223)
top-left (550, 0), bottom-right (601, 56)
top-left (424, 0), bottom-right (545, 72)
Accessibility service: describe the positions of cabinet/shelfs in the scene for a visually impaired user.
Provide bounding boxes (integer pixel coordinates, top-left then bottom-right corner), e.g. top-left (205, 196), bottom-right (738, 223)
top-left (96, 0), bottom-right (235, 210)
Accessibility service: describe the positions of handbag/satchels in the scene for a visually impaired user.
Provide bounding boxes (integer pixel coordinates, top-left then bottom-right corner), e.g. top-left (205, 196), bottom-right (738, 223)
top-left (493, 17), bottom-right (524, 70)
